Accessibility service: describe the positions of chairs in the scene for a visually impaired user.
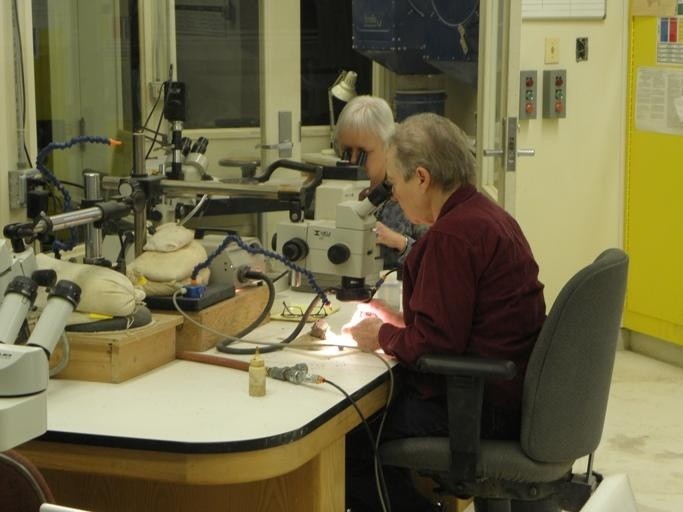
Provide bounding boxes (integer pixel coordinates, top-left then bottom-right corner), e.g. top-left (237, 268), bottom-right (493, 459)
top-left (350, 247), bottom-right (630, 512)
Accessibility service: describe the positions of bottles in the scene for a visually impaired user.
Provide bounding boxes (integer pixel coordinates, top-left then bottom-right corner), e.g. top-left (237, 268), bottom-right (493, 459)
top-left (248, 344), bottom-right (267, 397)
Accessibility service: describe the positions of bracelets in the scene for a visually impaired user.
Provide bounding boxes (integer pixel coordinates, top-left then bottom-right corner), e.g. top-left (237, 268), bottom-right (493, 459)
top-left (395, 236), bottom-right (407, 257)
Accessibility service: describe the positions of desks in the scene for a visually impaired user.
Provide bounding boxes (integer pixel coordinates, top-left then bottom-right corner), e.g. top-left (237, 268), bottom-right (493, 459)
top-left (0, 269), bottom-right (404, 512)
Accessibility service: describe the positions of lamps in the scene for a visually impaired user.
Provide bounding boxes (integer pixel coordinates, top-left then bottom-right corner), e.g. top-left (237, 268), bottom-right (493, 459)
top-left (322, 70), bottom-right (358, 154)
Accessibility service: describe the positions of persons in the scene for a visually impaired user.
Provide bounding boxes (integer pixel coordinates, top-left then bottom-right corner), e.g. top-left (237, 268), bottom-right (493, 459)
top-left (332, 94), bottom-right (430, 271)
top-left (346, 111), bottom-right (552, 512)
